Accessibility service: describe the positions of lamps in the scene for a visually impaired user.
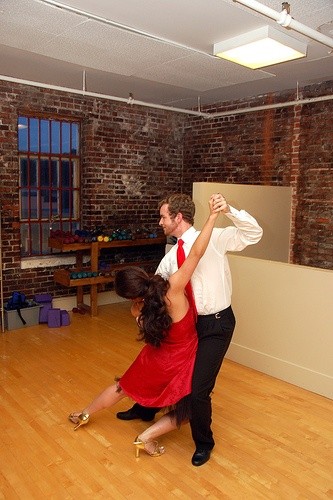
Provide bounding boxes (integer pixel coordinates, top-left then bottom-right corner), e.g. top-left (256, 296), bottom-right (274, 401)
top-left (213, 24), bottom-right (306, 70)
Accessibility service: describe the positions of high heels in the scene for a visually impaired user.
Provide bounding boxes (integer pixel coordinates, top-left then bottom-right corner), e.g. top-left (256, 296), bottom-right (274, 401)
top-left (70, 411), bottom-right (90, 431)
top-left (132, 436), bottom-right (166, 458)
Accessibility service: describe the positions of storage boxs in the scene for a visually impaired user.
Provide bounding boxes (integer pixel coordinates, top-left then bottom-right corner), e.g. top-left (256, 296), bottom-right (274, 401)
top-left (5, 303), bottom-right (43, 330)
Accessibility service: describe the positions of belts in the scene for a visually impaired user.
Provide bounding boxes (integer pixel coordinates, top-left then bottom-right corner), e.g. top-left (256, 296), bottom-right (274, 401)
top-left (197, 306), bottom-right (232, 320)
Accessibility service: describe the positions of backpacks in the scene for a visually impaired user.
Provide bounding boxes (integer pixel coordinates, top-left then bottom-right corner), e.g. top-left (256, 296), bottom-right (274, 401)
top-left (6, 292), bottom-right (28, 325)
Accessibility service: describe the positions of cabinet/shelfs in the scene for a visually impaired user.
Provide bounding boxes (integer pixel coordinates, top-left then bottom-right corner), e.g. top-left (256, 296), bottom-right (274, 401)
top-left (49, 231), bottom-right (165, 317)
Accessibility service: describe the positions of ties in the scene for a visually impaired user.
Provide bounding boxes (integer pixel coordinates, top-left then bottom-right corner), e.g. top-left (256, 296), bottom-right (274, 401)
top-left (177, 239), bottom-right (197, 323)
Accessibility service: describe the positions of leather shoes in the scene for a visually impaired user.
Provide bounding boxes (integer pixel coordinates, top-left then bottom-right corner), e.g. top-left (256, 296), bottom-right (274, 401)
top-left (192, 447), bottom-right (210, 466)
top-left (117, 409), bottom-right (154, 422)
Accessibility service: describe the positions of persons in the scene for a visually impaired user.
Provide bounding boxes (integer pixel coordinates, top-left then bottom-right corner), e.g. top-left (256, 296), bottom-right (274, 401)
top-left (68, 195), bottom-right (220, 458)
top-left (136, 193), bottom-right (263, 466)
